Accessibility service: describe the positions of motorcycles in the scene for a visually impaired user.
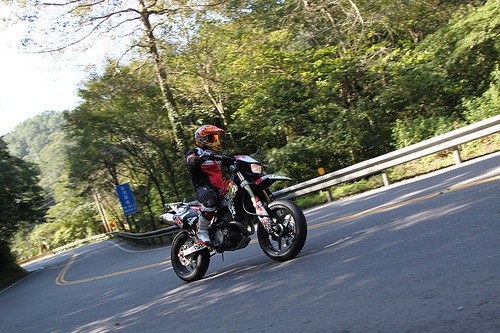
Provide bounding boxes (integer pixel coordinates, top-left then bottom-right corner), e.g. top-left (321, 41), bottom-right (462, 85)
top-left (158, 153), bottom-right (308, 283)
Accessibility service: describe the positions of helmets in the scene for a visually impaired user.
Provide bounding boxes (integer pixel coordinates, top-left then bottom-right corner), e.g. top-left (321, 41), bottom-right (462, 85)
top-left (195, 124), bottom-right (225, 151)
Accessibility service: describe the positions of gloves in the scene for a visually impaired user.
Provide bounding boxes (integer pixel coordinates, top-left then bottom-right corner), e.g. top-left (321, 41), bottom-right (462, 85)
top-left (199, 149), bottom-right (218, 161)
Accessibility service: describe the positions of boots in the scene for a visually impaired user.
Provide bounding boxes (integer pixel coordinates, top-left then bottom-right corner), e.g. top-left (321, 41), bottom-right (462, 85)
top-left (196, 209), bottom-right (212, 246)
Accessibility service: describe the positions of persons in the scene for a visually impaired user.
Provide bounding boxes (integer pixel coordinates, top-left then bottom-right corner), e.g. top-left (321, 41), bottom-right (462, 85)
top-left (186, 125), bottom-right (226, 246)
